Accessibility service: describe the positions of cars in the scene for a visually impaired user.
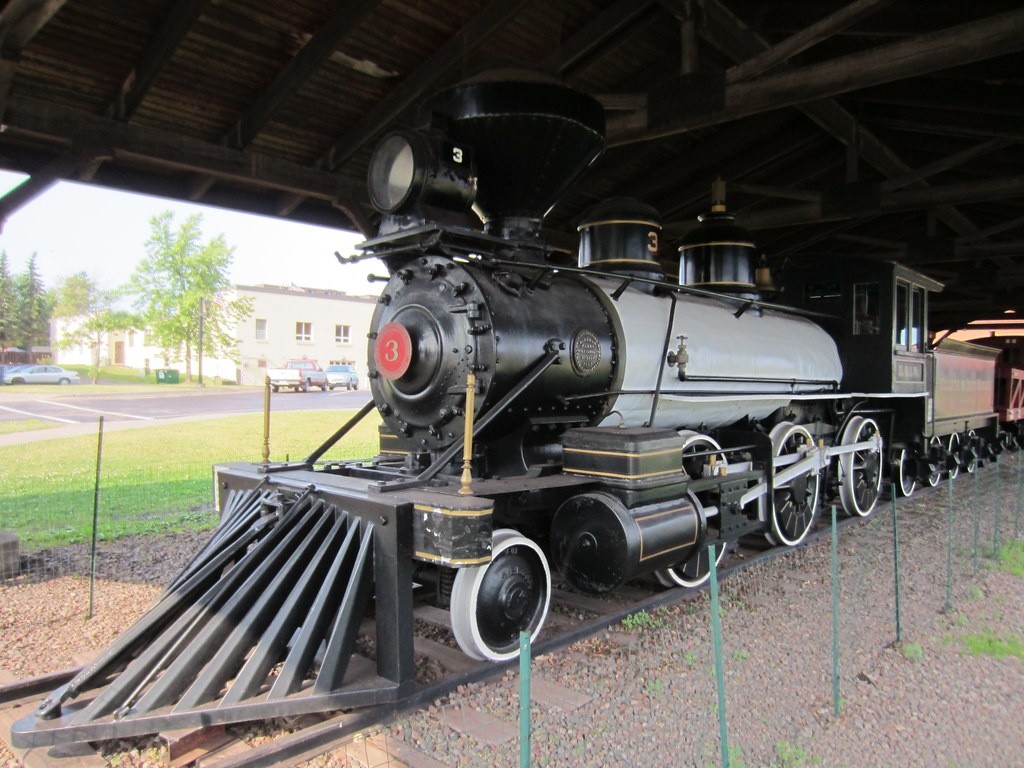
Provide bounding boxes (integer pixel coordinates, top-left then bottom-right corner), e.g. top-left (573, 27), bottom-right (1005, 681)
top-left (324, 364), bottom-right (360, 390)
top-left (4, 365), bottom-right (81, 385)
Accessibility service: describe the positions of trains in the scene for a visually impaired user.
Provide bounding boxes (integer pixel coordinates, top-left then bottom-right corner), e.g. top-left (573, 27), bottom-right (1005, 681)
top-left (10, 79), bottom-right (1024, 748)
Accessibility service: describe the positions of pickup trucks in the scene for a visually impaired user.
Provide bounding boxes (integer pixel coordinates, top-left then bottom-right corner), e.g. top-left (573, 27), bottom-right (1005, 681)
top-left (267, 358), bottom-right (328, 392)
